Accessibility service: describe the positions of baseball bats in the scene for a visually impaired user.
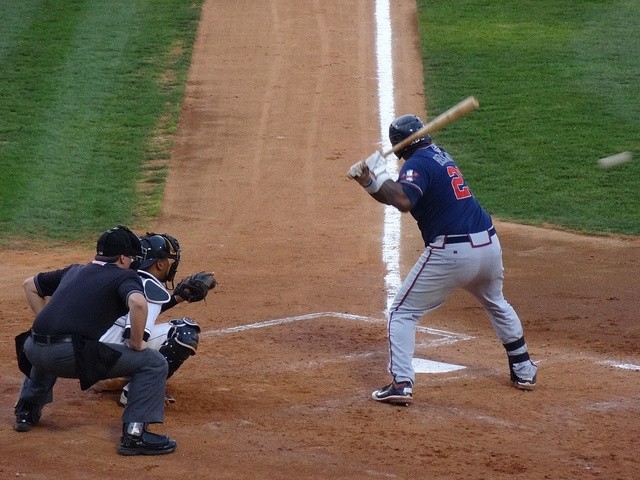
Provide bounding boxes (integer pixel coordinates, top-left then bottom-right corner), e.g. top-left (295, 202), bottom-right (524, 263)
top-left (347, 95), bottom-right (480, 180)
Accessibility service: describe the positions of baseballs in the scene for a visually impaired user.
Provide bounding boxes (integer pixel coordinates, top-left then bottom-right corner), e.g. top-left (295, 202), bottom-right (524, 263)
top-left (597, 151), bottom-right (631, 170)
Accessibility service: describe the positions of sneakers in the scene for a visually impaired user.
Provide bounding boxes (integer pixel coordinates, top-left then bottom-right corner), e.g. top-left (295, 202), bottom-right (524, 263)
top-left (15, 409), bottom-right (35, 432)
top-left (515, 374), bottom-right (536, 390)
top-left (120, 390), bottom-right (175, 408)
top-left (372, 383), bottom-right (413, 403)
top-left (117, 435), bottom-right (176, 456)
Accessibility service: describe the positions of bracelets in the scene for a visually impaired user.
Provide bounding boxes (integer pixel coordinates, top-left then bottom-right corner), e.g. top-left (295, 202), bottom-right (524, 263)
top-left (362, 177), bottom-right (382, 194)
top-left (376, 172), bottom-right (392, 189)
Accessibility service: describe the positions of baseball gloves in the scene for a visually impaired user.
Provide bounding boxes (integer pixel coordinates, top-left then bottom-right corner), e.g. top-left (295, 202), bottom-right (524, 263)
top-left (173, 271), bottom-right (219, 303)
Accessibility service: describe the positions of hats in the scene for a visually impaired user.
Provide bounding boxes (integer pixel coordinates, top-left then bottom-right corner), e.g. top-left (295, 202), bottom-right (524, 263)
top-left (95, 225), bottom-right (142, 263)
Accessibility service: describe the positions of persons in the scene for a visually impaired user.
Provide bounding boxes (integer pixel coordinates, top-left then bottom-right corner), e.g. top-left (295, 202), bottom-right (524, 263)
top-left (348, 115), bottom-right (538, 402)
top-left (15, 227), bottom-right (175, 455)
top-left (99, 232), bottom-right (216, 409)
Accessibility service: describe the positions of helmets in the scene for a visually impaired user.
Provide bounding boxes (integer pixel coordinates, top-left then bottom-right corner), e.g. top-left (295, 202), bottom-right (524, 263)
top-left (389, 114), bottom-right (432, 160)
top-left (132, 232), bottom-right (181, 290)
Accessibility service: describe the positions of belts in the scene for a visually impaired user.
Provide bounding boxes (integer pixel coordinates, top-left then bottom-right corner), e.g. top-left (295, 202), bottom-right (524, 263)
top-left (30, 328), bottom-right (72, 344)
top-left (445, 226), bottom-right (496, 243)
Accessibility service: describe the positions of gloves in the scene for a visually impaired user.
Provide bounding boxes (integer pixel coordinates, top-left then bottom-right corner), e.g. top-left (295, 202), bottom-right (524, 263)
top-left (366, 150), bottom-right (392, 189)
top-left (348, 160), bottom-right (380, 195)
